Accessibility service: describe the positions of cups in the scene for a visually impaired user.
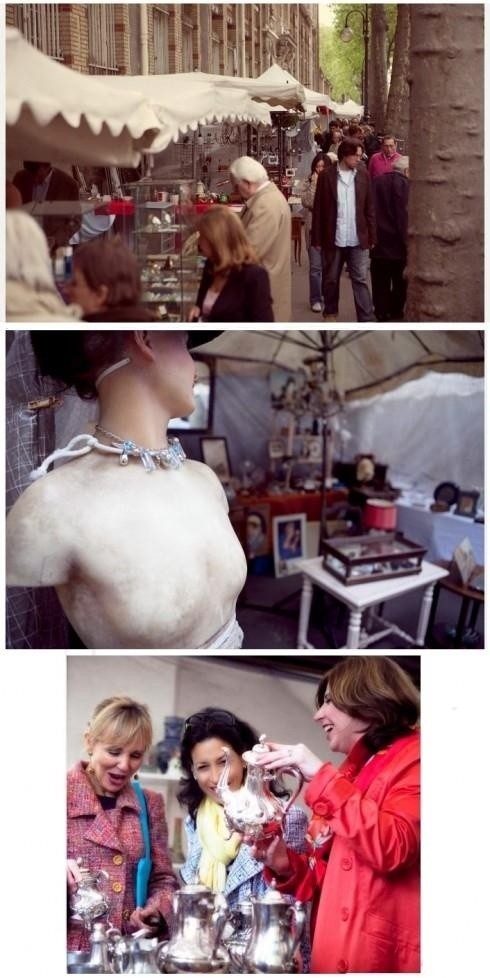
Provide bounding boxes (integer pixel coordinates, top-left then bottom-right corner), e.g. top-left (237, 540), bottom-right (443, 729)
top-left (157, 192), bottom-right (168, 203)
top-left (169, 193), bottom-right (180, 204)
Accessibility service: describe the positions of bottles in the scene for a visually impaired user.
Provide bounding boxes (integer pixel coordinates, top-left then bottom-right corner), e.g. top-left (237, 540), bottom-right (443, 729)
top-left (195, 179), bottom-right (205, 193)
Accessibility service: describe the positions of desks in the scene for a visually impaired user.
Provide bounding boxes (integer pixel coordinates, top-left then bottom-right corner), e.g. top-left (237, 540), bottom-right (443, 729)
top-left (219, 478), bottom-right (351, 568)
top-left (297, 547), bottom-right (450, 650)
top-left (377, 484), bottom-right (484, 636)
top-left (290, 212), bottom-right (305, 265)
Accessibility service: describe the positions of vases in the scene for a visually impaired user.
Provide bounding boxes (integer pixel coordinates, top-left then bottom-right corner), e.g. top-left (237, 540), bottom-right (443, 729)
top-left (169, 817), bottom-right (187, 864)
top-left (155, 715), bottom-right (187, 773)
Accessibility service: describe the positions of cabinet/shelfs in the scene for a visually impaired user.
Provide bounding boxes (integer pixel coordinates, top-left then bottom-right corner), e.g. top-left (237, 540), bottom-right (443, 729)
top-left (134, 771), bottom-right (195, 864)
top-left (132, 178), bottom-right (199, 322)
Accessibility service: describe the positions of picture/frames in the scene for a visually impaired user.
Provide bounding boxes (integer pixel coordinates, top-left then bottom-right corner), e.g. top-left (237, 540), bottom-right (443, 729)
top-left (271, 512), bottom-right (310, 578)
top-left (197, 437), bottom-right (234, 483)
top-left (455, 490), bottom-right (480, 517)
top-left (267, 438), bottom-right (287, 458)
top-left (168, 351), bottom-right (218, 436)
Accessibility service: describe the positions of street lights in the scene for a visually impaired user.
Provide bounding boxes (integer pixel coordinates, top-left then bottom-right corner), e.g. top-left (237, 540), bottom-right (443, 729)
top-left (339, 4), bottom-right (368, 126)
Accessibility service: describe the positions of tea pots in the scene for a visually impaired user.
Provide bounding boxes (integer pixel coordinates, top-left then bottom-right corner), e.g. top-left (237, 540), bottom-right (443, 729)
top-left (217, 733), bottom-right (305, 849)
top-left (152, 874), bottom-right (234, 973)
top-left (222, 895), bottom-right (256, 972)
top-left (242, 878), bottom-right (308, 973)
top-left (216, 191), bottom-right (230, 203)
top-left (79, 922), bottom-right (155, 973)
top-left (68, 865), bottom-right (112, 922)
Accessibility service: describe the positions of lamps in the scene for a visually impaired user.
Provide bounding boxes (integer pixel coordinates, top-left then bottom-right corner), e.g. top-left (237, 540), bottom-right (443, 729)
top-left (361, 499), bottom-right (398, 538)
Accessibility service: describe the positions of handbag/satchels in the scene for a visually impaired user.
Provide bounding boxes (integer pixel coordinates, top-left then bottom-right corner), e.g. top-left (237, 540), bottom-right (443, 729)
top-left (136, 858), bottom-right (154, 905)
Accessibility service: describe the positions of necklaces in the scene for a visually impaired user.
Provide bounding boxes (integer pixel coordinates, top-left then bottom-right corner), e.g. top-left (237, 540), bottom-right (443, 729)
top-left (92, 423), bottom-right (188, 472)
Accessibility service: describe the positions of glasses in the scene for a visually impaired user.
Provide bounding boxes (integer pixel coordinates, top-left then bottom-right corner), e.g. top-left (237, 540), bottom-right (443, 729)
top-left (184, 711), bottom-right (235, 731)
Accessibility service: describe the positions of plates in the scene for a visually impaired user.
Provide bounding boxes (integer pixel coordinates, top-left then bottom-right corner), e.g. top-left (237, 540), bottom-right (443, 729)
top-left (121, 196), bottom-right (134, 202)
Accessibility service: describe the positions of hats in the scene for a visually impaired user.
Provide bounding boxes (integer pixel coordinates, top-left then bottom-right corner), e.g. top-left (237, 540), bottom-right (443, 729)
top-left (32, 328), bottom-right (225, 374)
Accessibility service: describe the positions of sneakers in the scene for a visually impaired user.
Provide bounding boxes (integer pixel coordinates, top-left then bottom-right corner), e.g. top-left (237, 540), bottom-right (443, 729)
top-left (310, 302), bottom-right (322, 311)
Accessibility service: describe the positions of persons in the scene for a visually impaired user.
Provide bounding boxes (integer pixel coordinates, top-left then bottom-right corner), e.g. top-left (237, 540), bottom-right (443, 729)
top-left (311, 139), bottom-right (373, 321)
top-left (300, 154), bottom-right (336, 313)
top-left (229, 155), bottom-right (294, 322)
top-left (250, 655), bottom-right (421, 973)
top-left (68, 231), bottom-right (163, 322)
top-left (7, 330), bottom-right (250, 649)
top-left (368, 136), bottom-right (402, 174)
top-left (370, 156), bottom-right (411, 323)
top-left (12, 158), bottom-right (81, 252)
top-left (314, 116), bottom-right (375, 153)
top-left (280, 522), bottom-right (301, 560)
top-left (67, 695), bottom-right (183, 954)
top-left (185, 205), bottom-right (280, 322)
top-left (174, 705), bottom-right (310, 974)
top-left (6, 210), bottom-right (83, 321)
top-left (246, 511), bottom-right (265, 551)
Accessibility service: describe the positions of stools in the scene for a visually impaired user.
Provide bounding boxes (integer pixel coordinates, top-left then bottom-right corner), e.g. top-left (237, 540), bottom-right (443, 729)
top-left (428, 556), bottom-right (485, 649)
